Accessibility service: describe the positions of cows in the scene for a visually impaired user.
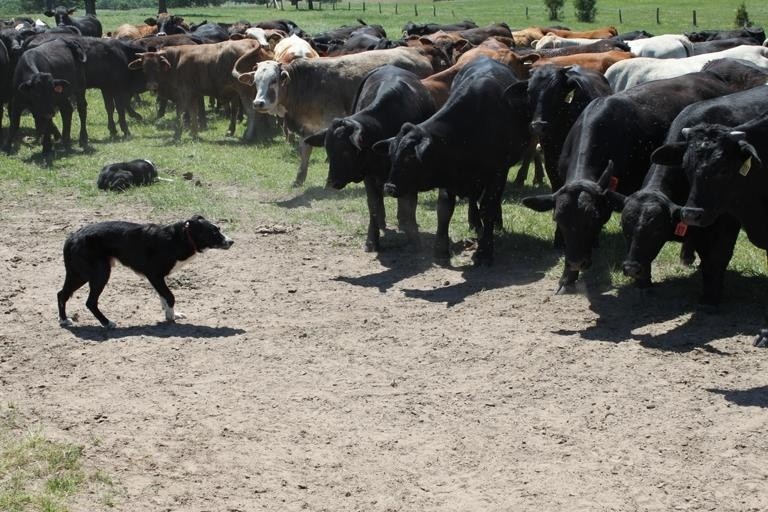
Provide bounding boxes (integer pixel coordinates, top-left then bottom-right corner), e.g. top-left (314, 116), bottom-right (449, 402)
top-left (237, 18), bottom-right (768, 347)
top-left (0, 6), bottom-right (321, 169)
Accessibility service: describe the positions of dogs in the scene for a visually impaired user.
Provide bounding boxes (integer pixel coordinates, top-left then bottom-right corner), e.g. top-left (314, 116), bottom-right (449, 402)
top-left (56, 214), bottom-right (235, 330)
top-left (96, 158), bottom-right (159, 194)
